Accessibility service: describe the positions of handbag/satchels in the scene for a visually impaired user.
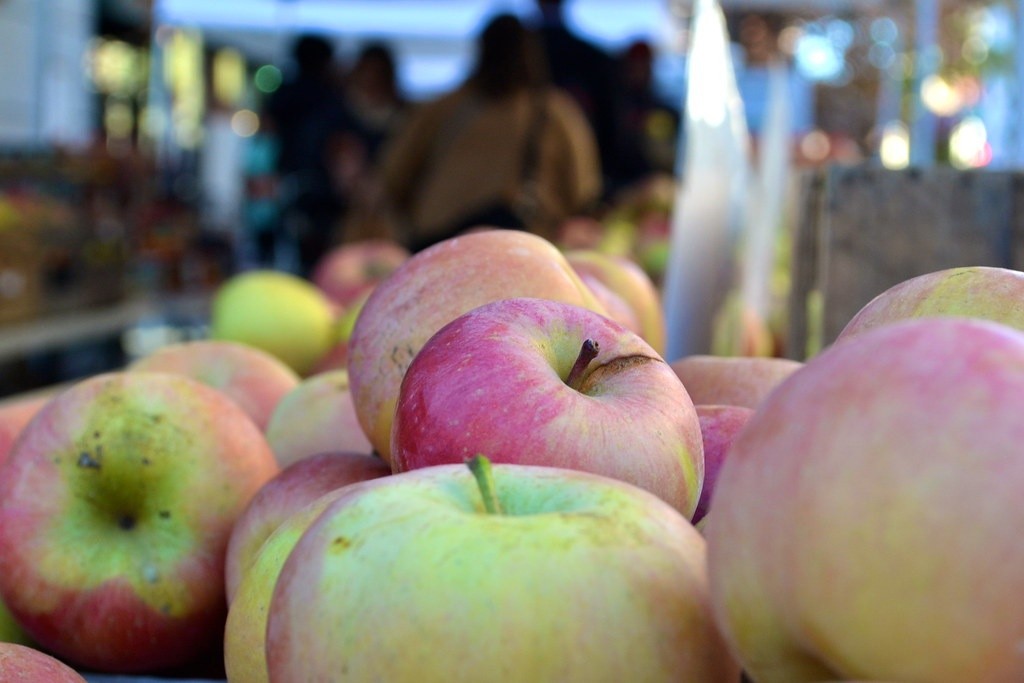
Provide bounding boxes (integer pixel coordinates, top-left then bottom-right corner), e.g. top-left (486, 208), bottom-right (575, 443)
top-left (407, 201), bottom-right (525, 254)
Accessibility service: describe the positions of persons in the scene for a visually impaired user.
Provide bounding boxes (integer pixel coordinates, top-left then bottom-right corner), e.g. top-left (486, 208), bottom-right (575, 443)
top-left (253, 1), bottom-right (679, 283)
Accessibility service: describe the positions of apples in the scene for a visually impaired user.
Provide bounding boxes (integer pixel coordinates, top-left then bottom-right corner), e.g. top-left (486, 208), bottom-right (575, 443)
top-left (0, 229), bottom-right (1024, 683)
